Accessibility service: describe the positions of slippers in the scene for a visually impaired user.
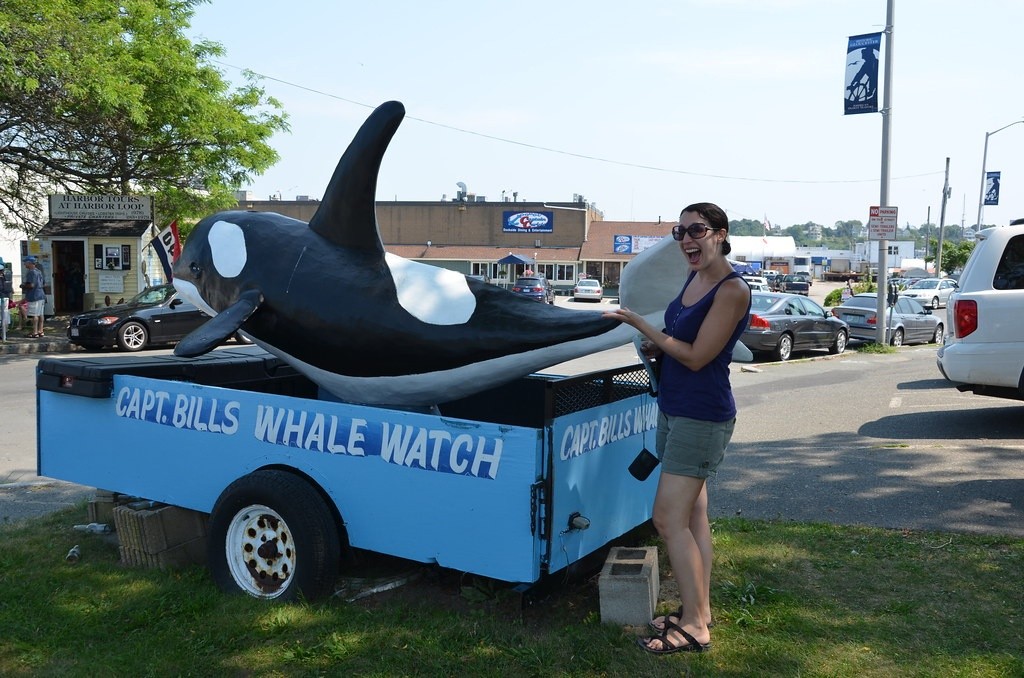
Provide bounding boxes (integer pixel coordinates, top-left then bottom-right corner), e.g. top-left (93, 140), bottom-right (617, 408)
top-left (637, 621), bottom-right (711, 654)
top-left (649, 605), bottom-right (714, 632)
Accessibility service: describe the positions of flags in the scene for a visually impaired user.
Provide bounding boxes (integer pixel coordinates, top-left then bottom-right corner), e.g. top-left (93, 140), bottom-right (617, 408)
top-left (150, 220), bottom-right (184, 284)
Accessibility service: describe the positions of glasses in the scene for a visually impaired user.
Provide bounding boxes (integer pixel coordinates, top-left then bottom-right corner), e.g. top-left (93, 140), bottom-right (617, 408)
top-left (672, 223), bottom-right (719, 241)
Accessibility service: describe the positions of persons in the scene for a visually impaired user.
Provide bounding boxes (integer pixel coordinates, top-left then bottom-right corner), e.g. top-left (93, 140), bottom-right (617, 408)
top-left (19, 257), bottom-right (46, 338)
top-left (603, 202), bottom-right (751, 654)
top-left (0, 263), bottom-right (13, 340)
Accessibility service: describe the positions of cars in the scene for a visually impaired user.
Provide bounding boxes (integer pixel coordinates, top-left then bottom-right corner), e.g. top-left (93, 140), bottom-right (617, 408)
top-left (574, 279), bottom-right (603, 302)
top-left (886, 276), bottom-right (959, 310)
top-left (937, 218), bottom-right (1023, 404)
top-left (831, 290), bottom-right (944, 347)
top-left (66, 284), bottom-right (257, 352)
top-left (739, 291), bottom-right (851, 362)
top-left (513, 277), bottom-right (554, 306)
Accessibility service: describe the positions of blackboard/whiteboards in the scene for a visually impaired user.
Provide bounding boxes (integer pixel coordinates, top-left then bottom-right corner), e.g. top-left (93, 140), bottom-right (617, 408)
top-left (98, 274), bottom-right (124, 293)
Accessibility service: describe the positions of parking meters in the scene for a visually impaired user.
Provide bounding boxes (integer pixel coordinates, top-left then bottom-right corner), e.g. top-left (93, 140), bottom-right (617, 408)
top-left (886, 280), bottom-right (902, 347)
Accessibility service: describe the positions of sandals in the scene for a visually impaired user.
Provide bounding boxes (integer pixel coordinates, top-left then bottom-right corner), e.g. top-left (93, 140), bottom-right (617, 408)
top-left (25, 332), bottom-right (39, 338)
top-left (39, 332), bottom-right (44, 337)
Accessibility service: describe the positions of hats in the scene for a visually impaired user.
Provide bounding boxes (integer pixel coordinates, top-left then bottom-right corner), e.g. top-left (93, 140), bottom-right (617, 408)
top-left (22, 255), bottom-right (36, 263)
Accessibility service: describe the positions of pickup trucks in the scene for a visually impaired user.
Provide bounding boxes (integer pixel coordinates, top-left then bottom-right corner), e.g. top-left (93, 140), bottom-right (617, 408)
top-left (741, 269), bottom-right (814, 297)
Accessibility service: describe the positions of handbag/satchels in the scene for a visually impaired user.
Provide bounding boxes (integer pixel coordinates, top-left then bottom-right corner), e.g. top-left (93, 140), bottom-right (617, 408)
top-left (628, 448), bottom-right (659, 481)
